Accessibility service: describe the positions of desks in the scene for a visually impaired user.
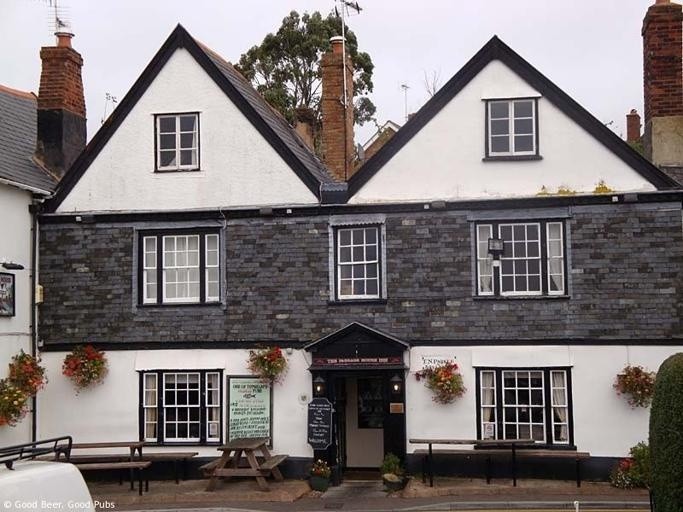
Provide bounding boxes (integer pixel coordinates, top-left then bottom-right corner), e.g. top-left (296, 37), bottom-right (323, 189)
top-left (204, 435), bottom-right (284, 492)
top-left (409, 439), bottom-right (534, 487)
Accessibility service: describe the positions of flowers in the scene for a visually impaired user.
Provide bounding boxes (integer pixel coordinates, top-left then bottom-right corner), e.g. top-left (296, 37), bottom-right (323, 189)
top-left (309, 460), bottom-right (330, 477)
top-left (0, 349), bottom-right (47, 425)
top-left (62, 342), bottom-right (108, 397)
top-left (614, 365), bottom-right (656, 410)
top-left (309, 476), bottom-right (329, 491)
top-left (607, 458), bottom-right (641, 490)
top-left (248, 344), bottom-right (288, 386)
top-left (415, 357), bottom-right (466, 406)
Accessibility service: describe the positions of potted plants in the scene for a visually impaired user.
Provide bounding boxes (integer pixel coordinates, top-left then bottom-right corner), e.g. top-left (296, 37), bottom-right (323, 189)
top-left (380, 451), bottom-right (409, 492)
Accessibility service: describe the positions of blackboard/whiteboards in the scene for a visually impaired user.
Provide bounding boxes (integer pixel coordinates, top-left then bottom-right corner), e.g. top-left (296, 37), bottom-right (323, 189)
top-left (307, 398), bottom-right (332, 450)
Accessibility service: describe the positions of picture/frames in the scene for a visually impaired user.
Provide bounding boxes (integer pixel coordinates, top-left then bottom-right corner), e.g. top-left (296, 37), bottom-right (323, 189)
top-left (225, 374), bottom-right (273, 450)
top-left (0, 272), bottom-right (15, 317)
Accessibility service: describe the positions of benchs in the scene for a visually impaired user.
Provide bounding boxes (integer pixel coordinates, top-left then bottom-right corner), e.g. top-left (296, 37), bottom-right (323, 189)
top-left (412, 448), bottom-right (589, 488)
top-left (36, 452), bottom-right (198, 497)
top-left (56, 441), bottom-right (146, 490)
top-left (198, 454), bottom-right (289, 471)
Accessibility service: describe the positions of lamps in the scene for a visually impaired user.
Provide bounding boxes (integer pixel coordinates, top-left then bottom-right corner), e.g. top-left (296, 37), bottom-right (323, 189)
top-left (388, 371), bottom-right (401, 395)
top-left (312, 375), bottom-right (325, 395)
